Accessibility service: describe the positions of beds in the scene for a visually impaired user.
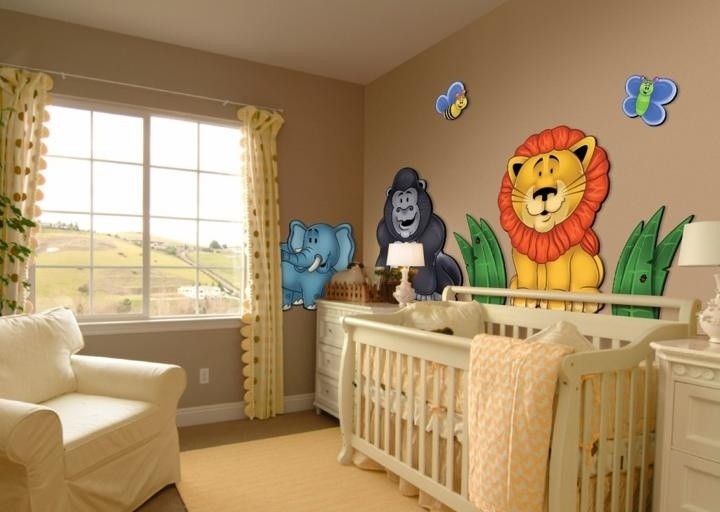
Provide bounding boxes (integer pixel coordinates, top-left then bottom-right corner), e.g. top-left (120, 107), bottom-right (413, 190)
top-left (336, 286), bottom-right (702, 512)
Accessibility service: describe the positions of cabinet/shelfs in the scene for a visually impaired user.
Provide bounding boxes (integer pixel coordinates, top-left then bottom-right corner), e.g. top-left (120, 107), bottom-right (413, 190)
top-left (649, 339), bottom-right (719, 511)
top-left (313, 298), bottom-right (403, 420)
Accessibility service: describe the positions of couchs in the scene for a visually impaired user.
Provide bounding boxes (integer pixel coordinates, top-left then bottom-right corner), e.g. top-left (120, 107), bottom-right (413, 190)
top-left (0, 305), bottom-right (187, 512)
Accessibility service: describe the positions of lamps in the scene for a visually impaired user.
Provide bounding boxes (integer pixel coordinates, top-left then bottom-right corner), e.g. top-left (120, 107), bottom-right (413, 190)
top-left (678, 220), bottom-right (719, 348)
top-left (386, 242), bottom-right (425, 307)
